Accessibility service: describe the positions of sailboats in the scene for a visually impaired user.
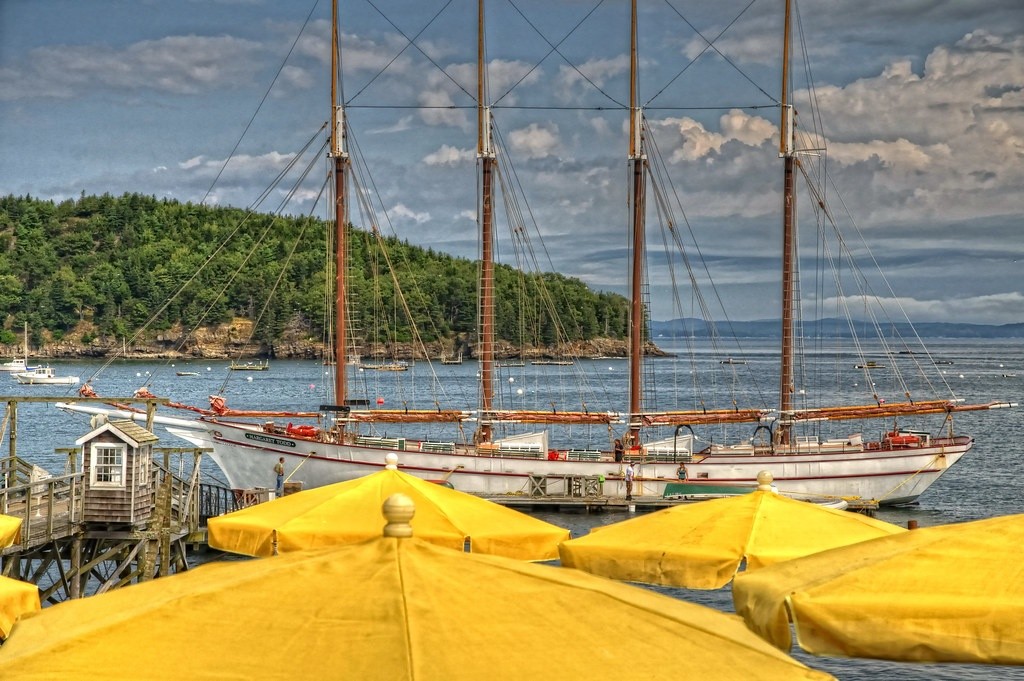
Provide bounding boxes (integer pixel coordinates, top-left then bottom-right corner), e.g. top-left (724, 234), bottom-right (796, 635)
top-left (0, 321), bottom-right (50, 371)
top-left (53, 1), bottom-right (1020, 512)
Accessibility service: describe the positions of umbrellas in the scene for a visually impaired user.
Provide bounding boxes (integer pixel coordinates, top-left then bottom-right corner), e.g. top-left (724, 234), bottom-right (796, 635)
top-left (207, 453), bottom-right (573, 563)
top-left (0, 575), bottom-right (42, 641)
top-left (0, 492), bottom-right (839, 681)
top-left (0, 513), bottom-right (24, 550)
top-left (731, 514), bottom-right (1024, 667)
top-left (557, 470), bottom-right (910, 591)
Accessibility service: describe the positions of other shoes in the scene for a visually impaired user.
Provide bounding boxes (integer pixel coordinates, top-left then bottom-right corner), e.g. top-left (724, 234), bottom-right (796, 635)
top-left (625, 495), bottom-right (635, 501)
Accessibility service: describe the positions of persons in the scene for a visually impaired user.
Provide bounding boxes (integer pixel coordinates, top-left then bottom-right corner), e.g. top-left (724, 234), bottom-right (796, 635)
top-left (614, 439), bottom-right (624, 461)
top-left (625, 460), bottom-right (636, 500)
top-left (273, 457), bottom-right (285, 497)
top-left (676, 462), bottom-right (689, 482)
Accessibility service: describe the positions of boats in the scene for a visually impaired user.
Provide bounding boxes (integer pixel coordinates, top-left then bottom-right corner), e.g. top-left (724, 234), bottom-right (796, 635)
top-left (387, 366), bottom-right (408, 372)
top-left (853, 363), bottom-right (887, 369)
top-left (358, 364), bottom-right (384, 369)
top-left (17, 368), bottom-right (79, 385)
top-left (323, 361), bottom-right (355, 365)
top-left (494, 362), bottom-right (525, 368)
top-left (9, 372), bottom-right (17, 379)
top-left (229, 359), bottom-right (270, 371)
top-left (1003, 373), bottom-right (1016, 377)
top-left (719, 359), bottom-right (749, 365)
top-left (933, 360), bottom-right (954, 365)
top-left (176, 371), bottom-right (200, 376)
top-left (440, 352), bottom-right (463, 365)
top-left (385, 361), bottom-right (409, 369)
top-left (531, 360), bottom-right (574, 367)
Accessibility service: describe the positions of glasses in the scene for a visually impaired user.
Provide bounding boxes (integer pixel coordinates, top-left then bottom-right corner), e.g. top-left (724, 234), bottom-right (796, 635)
top-left (632, 464), bottom-right (635, 466)
